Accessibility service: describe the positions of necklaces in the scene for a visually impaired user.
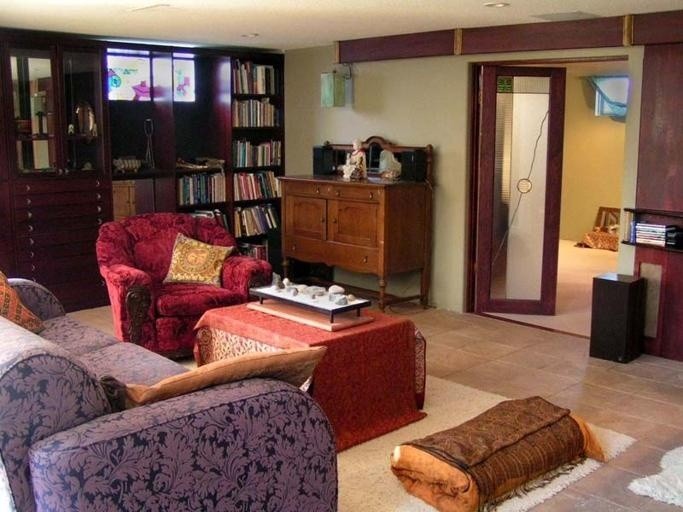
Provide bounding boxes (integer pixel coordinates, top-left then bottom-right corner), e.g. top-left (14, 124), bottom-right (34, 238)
top-left (351, 149), bottom-right (360, 156)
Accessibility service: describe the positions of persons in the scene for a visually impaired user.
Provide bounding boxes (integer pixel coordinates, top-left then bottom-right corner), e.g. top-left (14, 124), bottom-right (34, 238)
top-left (343, 140), bottom-right (367, 182)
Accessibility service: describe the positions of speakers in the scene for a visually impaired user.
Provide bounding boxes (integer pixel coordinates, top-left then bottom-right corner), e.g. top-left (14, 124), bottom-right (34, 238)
top-left (401, 150), bottom-right (427, 182)
top-left (590, 273), bottom-right (648, 363)
top-left (313, 145), bottom-right (333, 175)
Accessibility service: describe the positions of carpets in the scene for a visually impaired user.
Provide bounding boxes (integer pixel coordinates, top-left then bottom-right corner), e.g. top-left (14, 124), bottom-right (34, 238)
top-left (64, 304), bottom-right (639, 512)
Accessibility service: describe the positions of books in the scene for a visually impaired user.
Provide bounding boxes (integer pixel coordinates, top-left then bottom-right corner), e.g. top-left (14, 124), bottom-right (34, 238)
top-left (179, 58), bottom-right (282, 262)
top-left (628, 221), bottom-right (678, 251)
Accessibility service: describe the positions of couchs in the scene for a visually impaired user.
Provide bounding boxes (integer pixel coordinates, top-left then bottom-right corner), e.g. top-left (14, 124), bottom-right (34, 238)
top-left (0, 278), bottom-right (341, 511)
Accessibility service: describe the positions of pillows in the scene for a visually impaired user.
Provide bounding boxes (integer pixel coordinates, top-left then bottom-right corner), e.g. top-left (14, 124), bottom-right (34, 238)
top-left (164, 231), bottom-right (235, 290)
top-left (2, 268), bottom-right (48, 338)
top-left (127, 343), bottom-right (330, 400)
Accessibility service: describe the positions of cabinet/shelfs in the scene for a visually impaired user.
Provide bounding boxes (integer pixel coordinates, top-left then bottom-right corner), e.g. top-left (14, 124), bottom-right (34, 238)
top-left (0, 27), bottom-right (288, 315)
top-left (274, 136), bottom-right (434, 315)
top-left (617, 205), bottom-right (681, 257)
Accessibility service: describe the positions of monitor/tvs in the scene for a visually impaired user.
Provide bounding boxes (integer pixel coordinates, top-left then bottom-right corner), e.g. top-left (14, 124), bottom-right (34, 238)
top-left (106, 48), bottom-right (199, 105)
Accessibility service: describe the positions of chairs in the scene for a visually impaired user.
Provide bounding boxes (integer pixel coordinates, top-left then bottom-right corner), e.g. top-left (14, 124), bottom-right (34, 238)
top-left (96, 210), bottom-right (275, 360)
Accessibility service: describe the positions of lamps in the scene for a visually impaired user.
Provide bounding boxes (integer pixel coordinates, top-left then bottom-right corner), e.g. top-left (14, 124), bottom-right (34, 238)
top-left (317, 63), bottom-right (353, 110)
